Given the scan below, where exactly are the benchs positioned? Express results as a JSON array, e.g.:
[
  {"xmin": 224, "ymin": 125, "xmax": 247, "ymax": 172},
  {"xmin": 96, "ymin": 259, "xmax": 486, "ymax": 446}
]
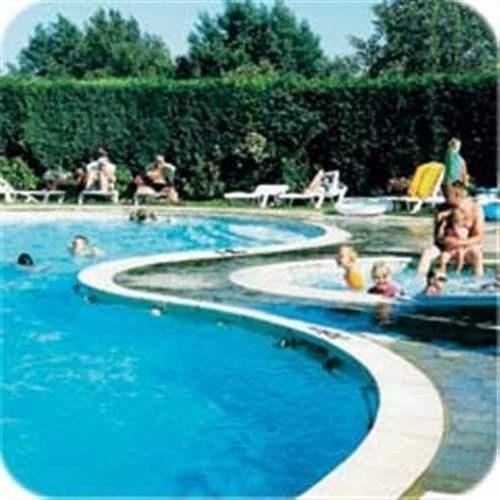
[
  {"xmin": 223, "ymin": 183, "xmax": 346, "ymax": 209},
  {"xmin": 1, "ymin": 172, "xmax": 118, "ymax": 206}
]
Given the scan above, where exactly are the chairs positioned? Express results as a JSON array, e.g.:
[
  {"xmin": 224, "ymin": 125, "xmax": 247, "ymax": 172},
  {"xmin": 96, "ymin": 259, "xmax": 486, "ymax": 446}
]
[{"xmin": 364, "ymin": 160, "xmax": 445, "ymax": 215}]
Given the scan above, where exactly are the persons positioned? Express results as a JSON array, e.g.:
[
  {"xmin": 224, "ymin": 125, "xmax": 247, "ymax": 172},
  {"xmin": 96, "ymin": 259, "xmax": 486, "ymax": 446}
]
[
  {"xmin": 415, "ymin": 179, "xmax": 486, "ymax": 275},
  {"xmin": 442, "ymin": 137, "xmax": 467, "ymax": 187},
  {"xmin": 83, "ymin": 148, "xmax": 118, "ymax": 192},
  {"xmin": 133, "ymin": 154, "xmax": 179, "ymax": 204},
  {"xmin": 129, "ymin": 208, "xmax": 158, "ymax": 224},
  {"xmin": 336, "ymin": 244, "xmax": 365, "ymax": 291},
  {"xmin": 434, "ymin": 209, "xmax": 472, "ymax": 276},
  {"xmin": 67, "ymin": 235, "xmax": 106, "ymax": 259},
  {"xmin": 420, "ymin": 266, "xmax": 449, "ymax": 298},
  {"xmin": 364, "ymin": 260, "xmax": 406, "ymax": 297}
]
